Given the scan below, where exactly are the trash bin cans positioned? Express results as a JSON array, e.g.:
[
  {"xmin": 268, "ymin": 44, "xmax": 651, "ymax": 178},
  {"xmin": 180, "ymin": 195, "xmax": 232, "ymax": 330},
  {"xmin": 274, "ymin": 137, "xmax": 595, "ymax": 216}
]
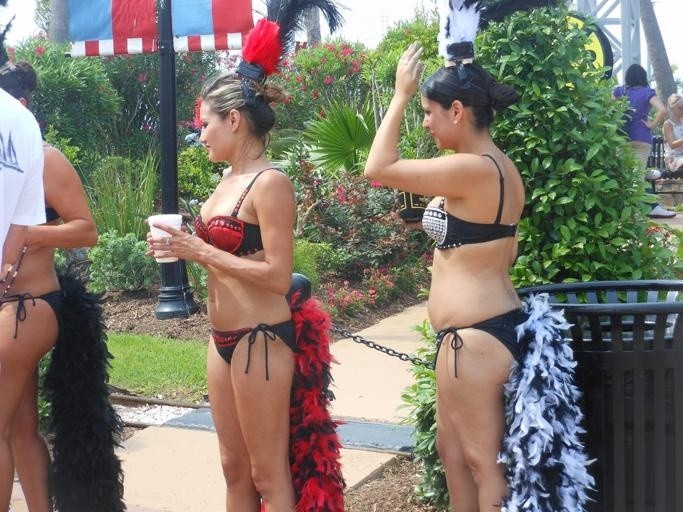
[{"xmin": 516, "ymin": 280, "xmax": 682, "ymax": 511}]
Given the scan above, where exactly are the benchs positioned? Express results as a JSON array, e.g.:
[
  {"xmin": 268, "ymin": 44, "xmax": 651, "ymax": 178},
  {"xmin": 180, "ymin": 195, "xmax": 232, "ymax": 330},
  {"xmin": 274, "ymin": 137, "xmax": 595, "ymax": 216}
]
[{"xmin": 646, "ymin": 135, "xmax": 683, "ymax": 195}]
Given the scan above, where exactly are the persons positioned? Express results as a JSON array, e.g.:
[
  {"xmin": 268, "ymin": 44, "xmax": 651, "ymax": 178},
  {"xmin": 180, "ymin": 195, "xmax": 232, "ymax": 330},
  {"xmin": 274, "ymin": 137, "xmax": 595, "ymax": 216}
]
[
  {"xmin": 361, "ymin": 41, "xmax": 530, "ymax": 512},
  {"xmin": 0, "ymin": 60, "xmax": 99, "ymax": 512},
  {"xmin": 0, "ymin": 87, "xmax": 46, "ymax": 292},
  {"xmin": 142, "ymin": 72, "xmax": 296, "ymax": 512},
  {"xmin": 610, "ymin": 63, "xmax": 664, "ymax": 196},
  {"xmin": 662, "ymin": 92, "xmax": 682, "ymax": 175}
]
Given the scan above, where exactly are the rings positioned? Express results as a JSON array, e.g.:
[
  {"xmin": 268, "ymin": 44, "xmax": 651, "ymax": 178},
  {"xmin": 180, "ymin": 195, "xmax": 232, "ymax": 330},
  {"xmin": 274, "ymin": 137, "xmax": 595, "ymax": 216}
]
[{"xmin": 165, "ymin": 236, "xmax": 171, "ymax": 247}]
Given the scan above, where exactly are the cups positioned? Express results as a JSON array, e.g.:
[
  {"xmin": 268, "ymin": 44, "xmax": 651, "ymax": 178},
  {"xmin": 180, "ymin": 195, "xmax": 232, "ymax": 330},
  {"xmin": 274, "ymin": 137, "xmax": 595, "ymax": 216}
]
[
  {"xmin": 397, "ymin": 188, "xmax": 426, "ymax": 223},
  {"xmin": 147, "ymin": 212, "xmax": 183, "ymax": 264}
]
